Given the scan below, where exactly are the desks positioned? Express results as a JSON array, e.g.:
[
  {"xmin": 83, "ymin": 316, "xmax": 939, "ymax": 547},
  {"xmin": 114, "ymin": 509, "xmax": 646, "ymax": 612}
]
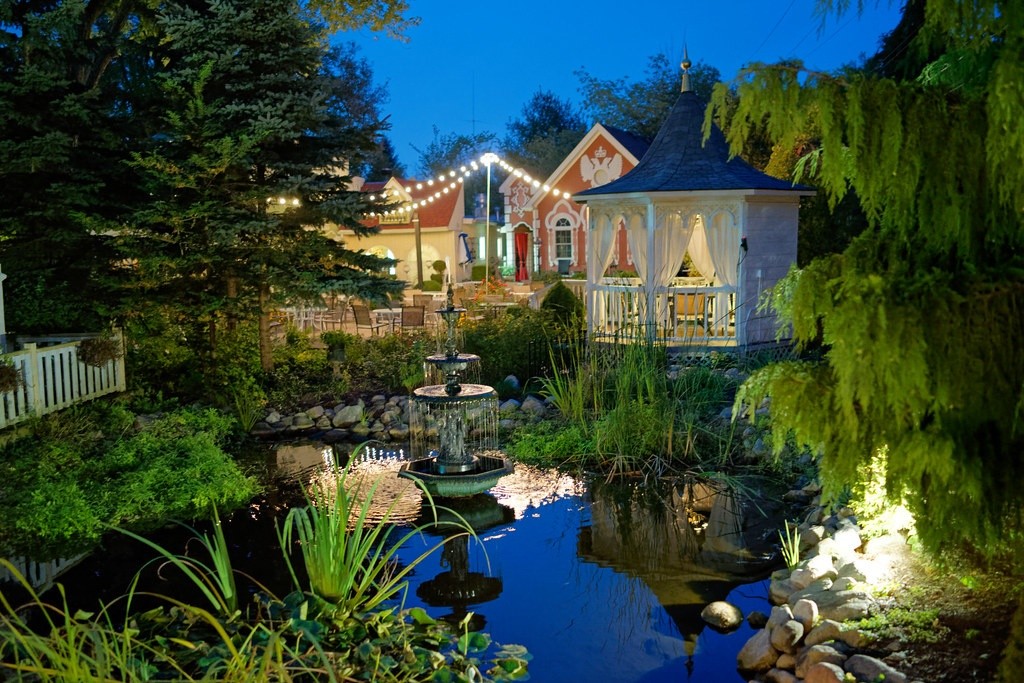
[
  {"xmin": 279, "ymin": 306, "xmax": 328, "ymax": 331},
  {"xmin": 480, "ymin": 302, "xmax": 518, "ymax": 319},
  {"xmin": 373, "ymin": 308, "xmax": 428, "ymax": 336}
]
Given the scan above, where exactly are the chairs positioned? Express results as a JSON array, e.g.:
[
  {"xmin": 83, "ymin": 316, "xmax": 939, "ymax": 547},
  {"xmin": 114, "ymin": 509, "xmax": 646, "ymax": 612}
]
[
  {"xmin": 668, "ymin": 287, "xmax": 714, "ymax": 338},
  {"xmin": 351, "ymin": 294, "xmax": 529, "ymax": 338},
  {"xmin": 269, "ymin": 294, "xmax": 355, "ymax": 336}
]
